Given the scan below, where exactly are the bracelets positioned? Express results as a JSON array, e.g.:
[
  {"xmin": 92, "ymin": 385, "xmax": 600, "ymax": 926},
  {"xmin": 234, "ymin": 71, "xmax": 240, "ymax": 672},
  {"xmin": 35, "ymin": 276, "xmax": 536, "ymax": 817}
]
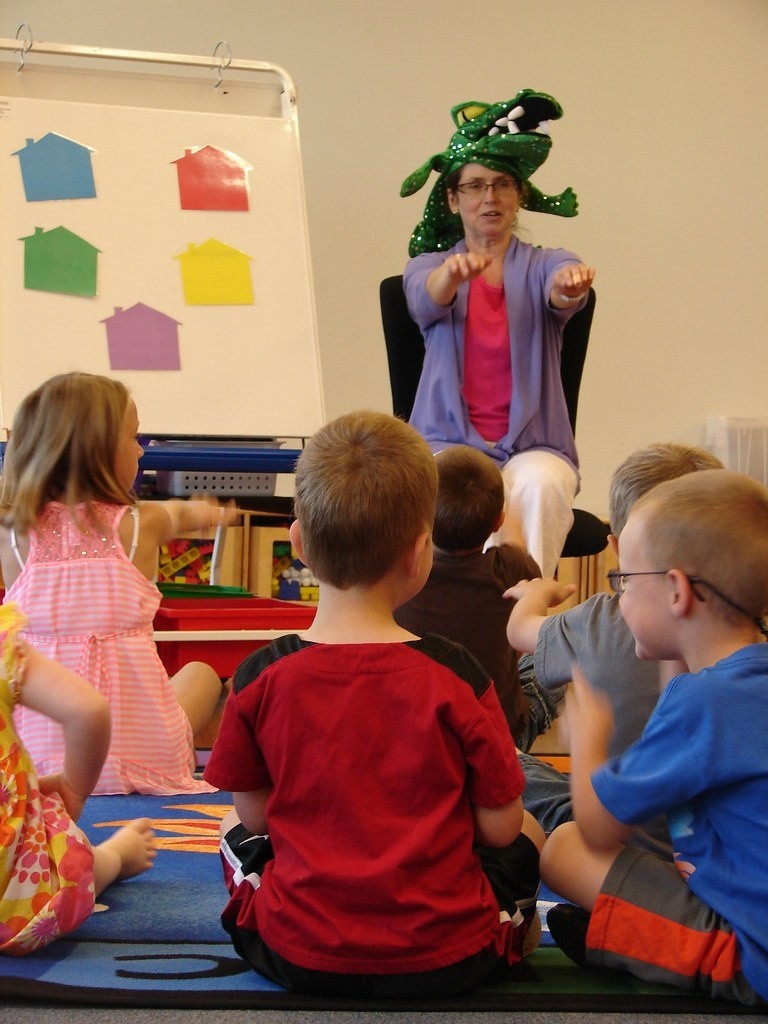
[{"xmin": 560, "ymin": 294, "xmax": 585, "ymax": 302}]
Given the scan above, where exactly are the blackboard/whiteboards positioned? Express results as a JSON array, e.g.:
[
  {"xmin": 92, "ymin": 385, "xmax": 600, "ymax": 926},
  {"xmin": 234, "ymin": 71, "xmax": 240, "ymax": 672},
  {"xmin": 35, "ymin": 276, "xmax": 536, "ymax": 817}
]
[{"xmin": 0, "ymin": 97, "xmax": 328, "ymax": 439}]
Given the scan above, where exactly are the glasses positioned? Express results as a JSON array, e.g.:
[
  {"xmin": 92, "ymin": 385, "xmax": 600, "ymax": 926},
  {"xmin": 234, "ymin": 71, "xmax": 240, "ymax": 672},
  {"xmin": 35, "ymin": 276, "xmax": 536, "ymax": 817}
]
[
  {"xmin": 452, "ymin": 178, "xmax": 520, "ymax": 194},
  {"xmin": 605, "ymin": 566, "xmax": 706, "ymax": 605}
]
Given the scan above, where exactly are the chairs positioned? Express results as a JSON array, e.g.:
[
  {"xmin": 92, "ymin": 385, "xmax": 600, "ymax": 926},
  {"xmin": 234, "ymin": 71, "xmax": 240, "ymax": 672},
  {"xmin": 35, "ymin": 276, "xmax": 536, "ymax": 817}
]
[{"xmin": 380, "ymin": 274, "xmax": 611, "ymax": 557}]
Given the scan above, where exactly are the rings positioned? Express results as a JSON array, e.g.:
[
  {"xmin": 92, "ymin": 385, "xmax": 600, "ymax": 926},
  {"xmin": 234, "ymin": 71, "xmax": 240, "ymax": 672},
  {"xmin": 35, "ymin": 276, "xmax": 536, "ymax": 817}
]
[{"xmin": 456, "ymin": 254, "xmax": 461, "ymax": 260}]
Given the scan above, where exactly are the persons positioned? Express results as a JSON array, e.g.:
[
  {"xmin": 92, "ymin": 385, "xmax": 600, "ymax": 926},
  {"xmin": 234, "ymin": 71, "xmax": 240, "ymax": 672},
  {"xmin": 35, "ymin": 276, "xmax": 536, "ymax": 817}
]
[
  {"xmin": 401, "ymin": 157, "xmax": 595, "ymax": 580},
  {"xmin": 216, "ymin": 408, "xmax": 546, "ymax": 1012},
  {"xmin": 503, "ymin": 444, "xmax": 768, "ymax": 1012},
  {"xmin": 1, "ymin": 373, "xmax": 238, "ymax": 957}
]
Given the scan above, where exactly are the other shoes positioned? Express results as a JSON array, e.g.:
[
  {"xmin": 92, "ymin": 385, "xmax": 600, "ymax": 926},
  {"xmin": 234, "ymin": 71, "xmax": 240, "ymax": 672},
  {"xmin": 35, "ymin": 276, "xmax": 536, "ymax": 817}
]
[{"xmin": 546, "ymin": 902, "xmax": 634, "ymax": 986}]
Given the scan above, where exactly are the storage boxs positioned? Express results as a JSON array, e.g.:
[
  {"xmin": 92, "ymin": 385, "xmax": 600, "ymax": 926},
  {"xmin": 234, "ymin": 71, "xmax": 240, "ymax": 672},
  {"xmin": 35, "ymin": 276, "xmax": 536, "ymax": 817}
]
[
  {"xmin": 154, "ymin": 440, "xmax": 287, "ymax": 496},
  {"xmin": 154, "ymin": 595, "xmax": 319, "ymax": 678},
  {"xmin": 157, "ymin": 526, "xmax": 320, "ymax": 605}
]
